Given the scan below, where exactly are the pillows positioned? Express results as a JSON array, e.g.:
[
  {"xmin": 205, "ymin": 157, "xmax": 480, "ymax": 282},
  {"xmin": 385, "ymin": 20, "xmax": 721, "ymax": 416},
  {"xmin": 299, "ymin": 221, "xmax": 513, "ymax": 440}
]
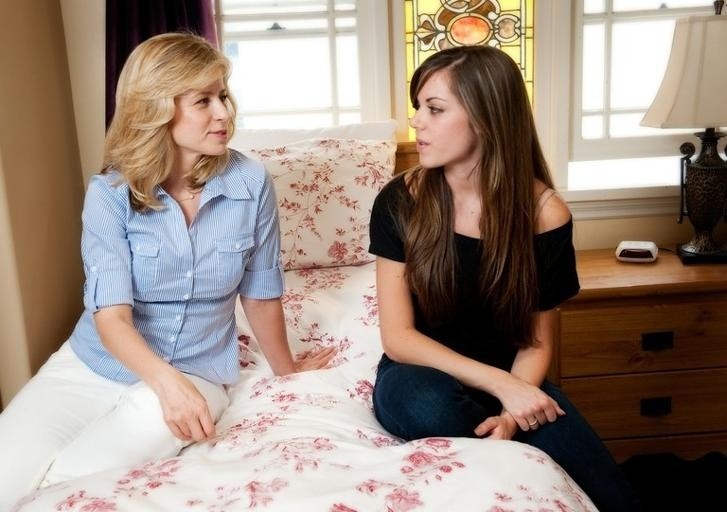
[{"xmin": 244, "ymin": 134, "xmax": 401, "ymax": 275}]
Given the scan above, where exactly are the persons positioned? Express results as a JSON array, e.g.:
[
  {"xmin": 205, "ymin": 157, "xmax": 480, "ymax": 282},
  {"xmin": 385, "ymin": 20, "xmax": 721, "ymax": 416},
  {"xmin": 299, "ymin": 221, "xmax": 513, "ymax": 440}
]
[
  {"xmin": 367, "ymin": 45, "xmax": 653, "ymax": 512},
  {"xmin": 0, "ymin": 33, "xmax": 337, "ymax": 512}
]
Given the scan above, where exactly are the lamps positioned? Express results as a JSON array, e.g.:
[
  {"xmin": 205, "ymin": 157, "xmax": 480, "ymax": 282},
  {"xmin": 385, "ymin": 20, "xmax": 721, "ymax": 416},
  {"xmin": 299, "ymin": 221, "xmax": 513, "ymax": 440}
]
[{"xmin": 630, "ymin": 0, "xmax": 727, "ymax": 267}]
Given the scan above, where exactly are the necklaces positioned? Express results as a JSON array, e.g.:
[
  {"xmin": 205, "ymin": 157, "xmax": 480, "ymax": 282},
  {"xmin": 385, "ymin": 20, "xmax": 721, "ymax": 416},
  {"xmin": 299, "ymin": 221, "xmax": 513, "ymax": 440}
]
[{"xmin": 160, "ymin": 182, "xmax": 195, "ymax": 203}]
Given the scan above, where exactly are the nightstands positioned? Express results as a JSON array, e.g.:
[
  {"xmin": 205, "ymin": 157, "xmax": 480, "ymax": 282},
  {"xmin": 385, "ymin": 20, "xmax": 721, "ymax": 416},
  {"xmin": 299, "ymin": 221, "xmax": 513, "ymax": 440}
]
[{"xmin": 541, "ymin": 241, "xmax": 726, "ymax": 467}]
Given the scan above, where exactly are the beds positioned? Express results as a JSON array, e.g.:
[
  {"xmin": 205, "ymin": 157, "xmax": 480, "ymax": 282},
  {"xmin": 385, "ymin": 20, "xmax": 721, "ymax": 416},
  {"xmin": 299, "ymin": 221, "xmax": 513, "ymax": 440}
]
[{"xmin": 51, "ymin": 140, "xmax": 593, "ymax": 512}]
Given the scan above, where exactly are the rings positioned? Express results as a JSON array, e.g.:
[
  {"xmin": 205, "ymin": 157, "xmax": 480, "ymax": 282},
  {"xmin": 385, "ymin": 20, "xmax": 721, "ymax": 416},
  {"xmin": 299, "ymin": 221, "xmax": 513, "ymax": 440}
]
[{"xmin": 529, "ymin": 419, "xmax": 538, "ymax": 426}]
